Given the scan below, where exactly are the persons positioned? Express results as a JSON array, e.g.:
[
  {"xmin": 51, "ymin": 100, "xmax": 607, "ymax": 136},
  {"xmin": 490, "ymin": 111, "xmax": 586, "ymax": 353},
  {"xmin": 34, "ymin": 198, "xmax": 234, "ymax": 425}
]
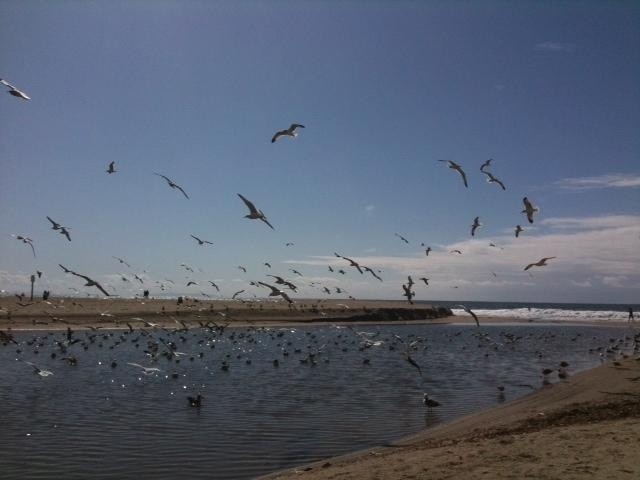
[{"xmin": 628, "ymin": 306, "xmax": 635, "ymax": 322}]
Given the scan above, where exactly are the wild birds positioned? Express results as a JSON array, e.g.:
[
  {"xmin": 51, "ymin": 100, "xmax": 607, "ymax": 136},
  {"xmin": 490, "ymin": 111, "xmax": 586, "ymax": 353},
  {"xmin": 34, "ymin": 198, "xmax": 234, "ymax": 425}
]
[
  {"xmin": 0, "ymin": 158, "xmax": 640, "ymax": 412},
  {"xmin": 0, "ymin": 79, "xmax": 31, "ymax": 100},
  {"xmin": 271, "ymin": 124, "xmax": 305, "ymax": 143}
]
